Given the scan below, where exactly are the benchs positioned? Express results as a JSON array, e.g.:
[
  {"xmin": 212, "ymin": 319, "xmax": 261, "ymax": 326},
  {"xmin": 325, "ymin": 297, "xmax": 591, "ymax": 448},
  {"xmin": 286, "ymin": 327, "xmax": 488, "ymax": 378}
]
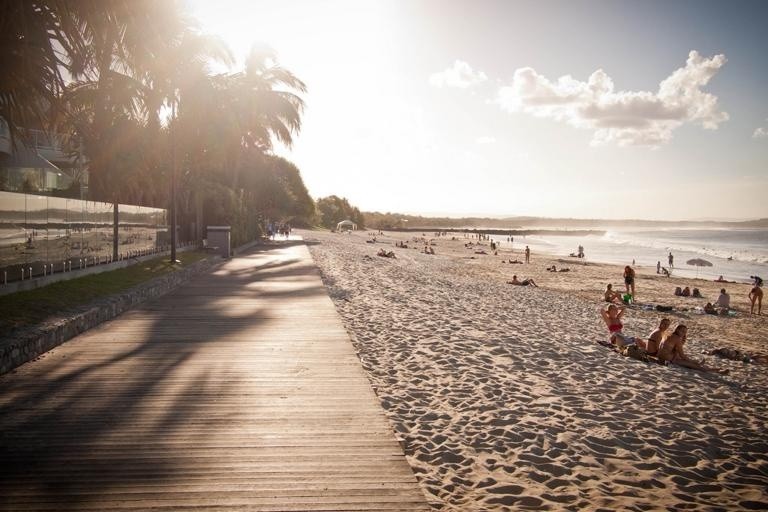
[{"xmin": 201, "ymin": 238, "xmax": 219, "ymax": 251}]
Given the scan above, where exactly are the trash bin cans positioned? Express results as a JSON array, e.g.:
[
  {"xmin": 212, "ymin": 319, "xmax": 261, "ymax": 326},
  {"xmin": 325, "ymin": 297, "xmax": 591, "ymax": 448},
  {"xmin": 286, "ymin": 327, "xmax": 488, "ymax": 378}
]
[{"xmin": 207, "ymin": 226, "xmax": 231, "ymax": 258}]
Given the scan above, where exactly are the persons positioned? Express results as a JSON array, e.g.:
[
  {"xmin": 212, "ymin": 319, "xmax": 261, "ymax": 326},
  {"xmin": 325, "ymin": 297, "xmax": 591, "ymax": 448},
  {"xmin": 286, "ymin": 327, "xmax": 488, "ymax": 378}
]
[
  {"xmin": 330, "ymin": 219, "xmax": 586, "ymax": 288},
  {"xmin": 603, "ymin": 250, "xmax": 765, "ymax": 315},
  {"xmin": 600, "ymin": 303, "xmax": 646, "ymax": 353},
  {"xmin": 645, "ymin": 318, "xmax": 670, "ymax": 357},
  {"xmin": 657, "ymin": 324, "xmax": 707, "ymax": 372},
  {"xmin": 257, "ymin": 216, "xmax": 292, "ymax": 244}
]
